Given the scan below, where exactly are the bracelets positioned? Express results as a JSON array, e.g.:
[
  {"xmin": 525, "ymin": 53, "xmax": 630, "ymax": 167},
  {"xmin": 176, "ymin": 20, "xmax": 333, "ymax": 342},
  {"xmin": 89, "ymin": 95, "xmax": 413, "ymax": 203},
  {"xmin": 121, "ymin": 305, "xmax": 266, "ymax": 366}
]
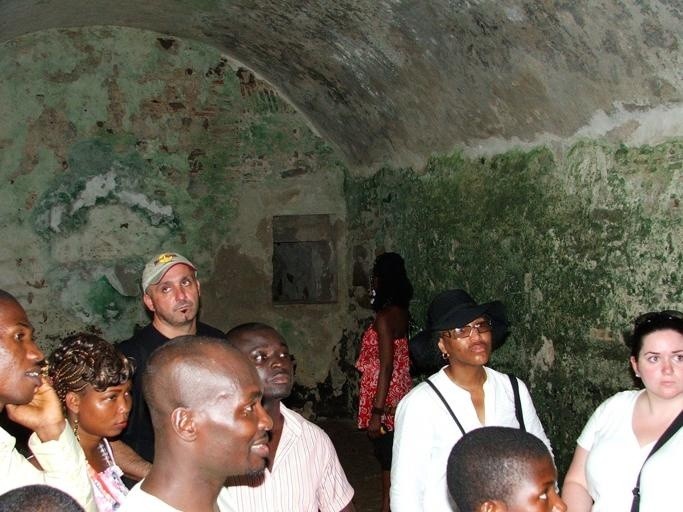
[{"xmin": 372, "ymin": 406, "xmax": 385, "ymax": 415}]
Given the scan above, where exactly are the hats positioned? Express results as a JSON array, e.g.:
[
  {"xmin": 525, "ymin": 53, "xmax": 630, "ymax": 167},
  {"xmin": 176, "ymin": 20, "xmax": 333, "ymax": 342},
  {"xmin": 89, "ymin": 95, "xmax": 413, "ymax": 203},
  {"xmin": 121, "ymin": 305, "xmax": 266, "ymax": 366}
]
[
  {"xmin": 141, "ymin": 253, "xmax": 198, "ymax": 294},
  {"xmin": 407, "ymin": 289, "xmax": 507, "ymax": 375}
]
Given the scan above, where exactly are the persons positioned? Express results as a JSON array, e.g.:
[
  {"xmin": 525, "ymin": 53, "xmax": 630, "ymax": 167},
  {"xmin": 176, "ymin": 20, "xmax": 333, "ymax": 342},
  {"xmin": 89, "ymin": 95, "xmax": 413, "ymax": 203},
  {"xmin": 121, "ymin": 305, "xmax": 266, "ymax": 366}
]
[
  {"xmin": 389, "ymin": 289, "xmax": 559, "ymax": 512},
  {"xmin": 447, "ymin": 425, "xmax": 569, "ymax": 512},
  {"xmin": 353, "ymin": 251, "xmax": 414, "ymax": 512},
  {"xmin": 559, "ymin": 310, "xmax": 683, "ymax": 512}
]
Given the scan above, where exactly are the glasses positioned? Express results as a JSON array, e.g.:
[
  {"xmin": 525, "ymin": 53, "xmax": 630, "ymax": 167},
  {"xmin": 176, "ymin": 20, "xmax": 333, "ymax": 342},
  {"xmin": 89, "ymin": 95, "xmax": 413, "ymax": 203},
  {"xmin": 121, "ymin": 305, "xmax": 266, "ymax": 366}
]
[
  {"xmin": 438, "ymin": 319, "xmax": 492, "ymax": 338},
  {"xmin": 634, "ymin": 310, "xmax": 683, "ymax": 335}
]
